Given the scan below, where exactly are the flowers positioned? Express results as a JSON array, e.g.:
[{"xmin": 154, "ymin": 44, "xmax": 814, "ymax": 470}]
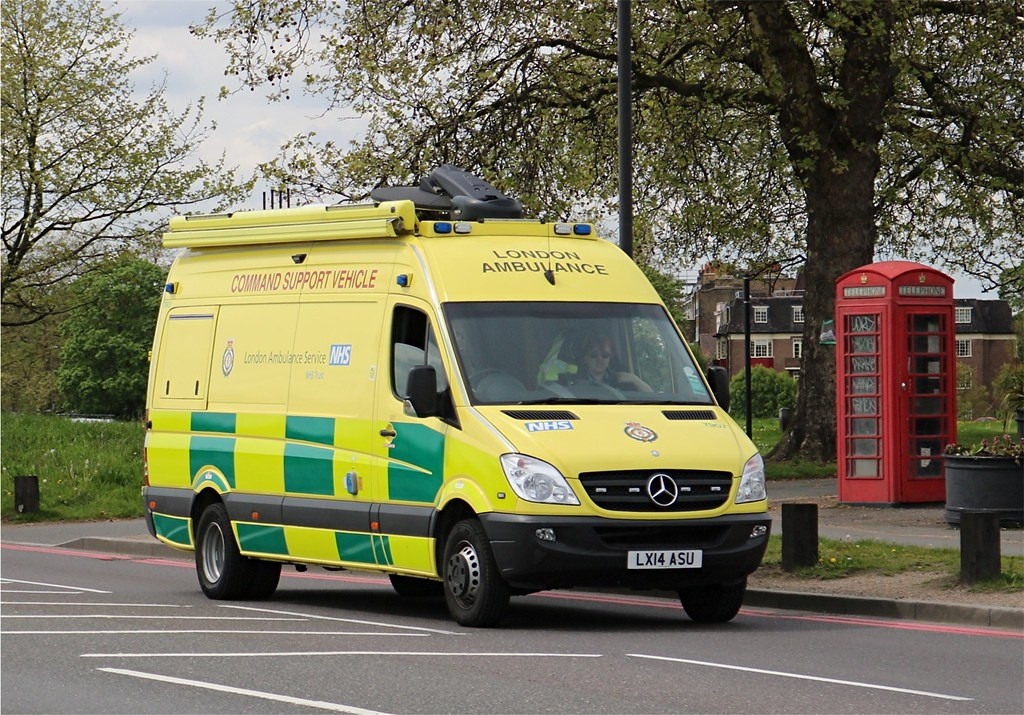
[{"xmin": 943, "ymin": 434, "xmax": 1024, "ymax": 464}]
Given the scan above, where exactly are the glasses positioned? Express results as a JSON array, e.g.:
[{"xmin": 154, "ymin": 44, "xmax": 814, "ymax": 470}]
[{"xmin": 591, "ymin": 351, "xmax": 612, "ymax": 359}]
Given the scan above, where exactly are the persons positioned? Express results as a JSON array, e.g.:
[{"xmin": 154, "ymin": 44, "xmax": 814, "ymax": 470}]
[
  {"xmin": 451, "ymin": 319, "xmax": 476, "ymax": 377},
  {"xmin": 568, "ymin": 334, "xmax": 654, "ymax": 392}
]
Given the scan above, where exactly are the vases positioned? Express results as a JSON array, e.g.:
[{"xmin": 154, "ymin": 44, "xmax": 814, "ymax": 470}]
[{"xmin": 941, "ymin": 453, "xmax": 1024, "ymax": 529}]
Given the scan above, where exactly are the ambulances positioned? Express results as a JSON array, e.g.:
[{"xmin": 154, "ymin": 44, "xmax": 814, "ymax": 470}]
[{"xmin": 139, "ymin": 166, "xmax": 774, "ymax": 627}]
